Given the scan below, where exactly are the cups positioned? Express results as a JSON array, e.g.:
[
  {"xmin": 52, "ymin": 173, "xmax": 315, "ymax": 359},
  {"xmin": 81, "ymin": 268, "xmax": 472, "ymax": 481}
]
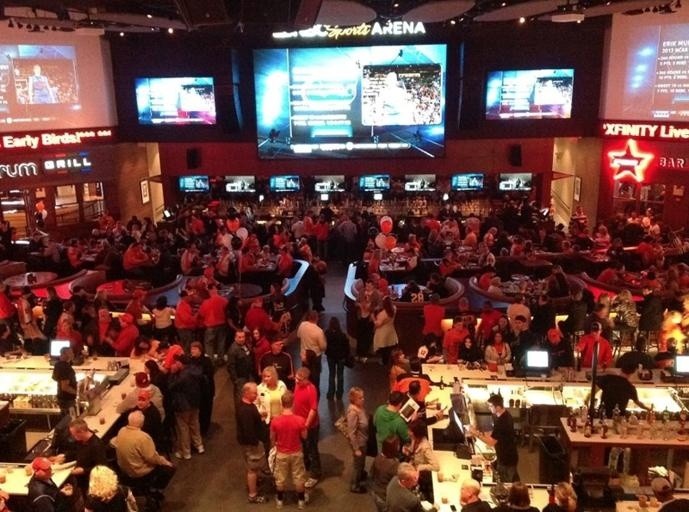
[{"xmin": 639, "ymin": 494, "xmax": 658, "ymax": 507}]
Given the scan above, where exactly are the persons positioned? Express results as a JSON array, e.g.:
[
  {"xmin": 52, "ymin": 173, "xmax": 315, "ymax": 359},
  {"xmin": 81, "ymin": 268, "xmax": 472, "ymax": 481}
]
[
  {"xmin": 26, "ymin": 63, "xmax": 54, "ymax": 105},
  {"xmin": 1, "ymin": 200, "xmax": 350, "ymax": 510},
  {"xmin": 346, "ymin": 199, "xmax": 689, "ymax": 512}
]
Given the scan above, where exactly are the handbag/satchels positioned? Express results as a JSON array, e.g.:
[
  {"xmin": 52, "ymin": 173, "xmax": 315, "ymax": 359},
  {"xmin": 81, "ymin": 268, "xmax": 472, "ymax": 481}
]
[
  {"xmin": 345, "ymin": 354, "xmax": 355, "ymax": 368},
  {"xmin": 333, "ymin": 415, "xmax": 358, "ymax": 440}
]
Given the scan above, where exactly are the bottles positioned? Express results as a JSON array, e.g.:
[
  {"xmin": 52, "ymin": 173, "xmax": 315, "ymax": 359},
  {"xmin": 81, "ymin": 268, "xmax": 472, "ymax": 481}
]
[
  {"xmin": 440, "ymin": 377, "xmax": 444, "ymax": 391},
  {"xmin": 549, "ymin": 484, "xmax": 555, "ymax": 504},
  {"xmin": 565, "ymin": 404, "xmax": 670, "ymax": 440}
]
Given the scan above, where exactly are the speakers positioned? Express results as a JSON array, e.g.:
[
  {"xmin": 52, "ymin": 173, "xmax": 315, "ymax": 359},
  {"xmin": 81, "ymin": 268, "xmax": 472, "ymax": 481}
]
[
  {"xmin": 509, "ymin": 144, "xmax": 521, "ymax": 164},
  {"xmin": 185, "ymin": 149, "xmax": 199, "ymax": 169}
]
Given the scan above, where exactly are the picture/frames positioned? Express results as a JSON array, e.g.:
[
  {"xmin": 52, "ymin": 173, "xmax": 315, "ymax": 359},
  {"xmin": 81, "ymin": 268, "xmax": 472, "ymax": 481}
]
[
  {"xmin": 139, "ymin": 179, "xmax": 151, "ymax": 205},
  {"xmin": 573, "ymin": 176, "xmax": 582, "ymax": 202}
]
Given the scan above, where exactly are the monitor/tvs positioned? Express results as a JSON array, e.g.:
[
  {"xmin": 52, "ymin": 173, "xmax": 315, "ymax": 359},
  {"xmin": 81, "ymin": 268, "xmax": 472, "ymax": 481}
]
[
  {"xmin": 498, "ymin": 173, "xmax": 534, "ymax": 192},
  {"xmin": 404, "ymin": 173, "xmax": 435, "ymax": 192},
  {"xmin": 672, "ymin": 354, "xmax": 689, "ymax": 376},
  {"xmin": 526, "ymin": 350, "xmax": 551, "ymax": 369},
  {"xmin": 225, "ymin": 174, "xmax": 255, "ymax": 195},
  {"xmin": 269, "ymin": 175, "xmax": 302, "ymax": 193},
  {"xmin": 450, "ymin": 173, "xmax": 485, "ymax": 193},
  {"xmin": 311, "ymin": 174, "xmax": 348, "ymax": 193},
  {"xmin": 449, "ymin": 410, "xmax": 464, "ymax": 440},
  {"xmin": 47, "ymin": 340, "xmax": 71, "ymax": 358},
  {"xmin": 357, "ymin": 174, "xmax": 392, "ymax": 190},
  {"xmin": 180, "ymin": 175, "xmax": 209, "ymax": 193}
]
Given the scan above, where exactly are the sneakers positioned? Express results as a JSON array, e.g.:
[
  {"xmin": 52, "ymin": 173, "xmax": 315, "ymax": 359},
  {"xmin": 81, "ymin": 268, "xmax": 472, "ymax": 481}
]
[
  {"xmin": 351, "ymin": 472, "xmax": 368, "ymax": 493},
  {"xmin": 175, "ymin": 452, "xmax": 191, "ymax": 460},
  {"xmin": 197, "ymin": 445, "xmax": 205, "ymax": 454},
  {"xmin": 248, "ymin": 469, "xmax": 318, "ymax": 509}
]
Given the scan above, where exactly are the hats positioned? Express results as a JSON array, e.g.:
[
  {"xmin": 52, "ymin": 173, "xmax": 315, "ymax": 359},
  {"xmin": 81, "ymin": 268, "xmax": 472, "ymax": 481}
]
[
  {"xmin": 174, "ymin": 354, "xmax": 190, "ymax": 365},
  {"xmin": 138, "ymin": 390, "xmax": 149, "ymax": 400},
  {"xmin": 652, "ymin": 477, "xmax": 673, "ymax": 493}
]
